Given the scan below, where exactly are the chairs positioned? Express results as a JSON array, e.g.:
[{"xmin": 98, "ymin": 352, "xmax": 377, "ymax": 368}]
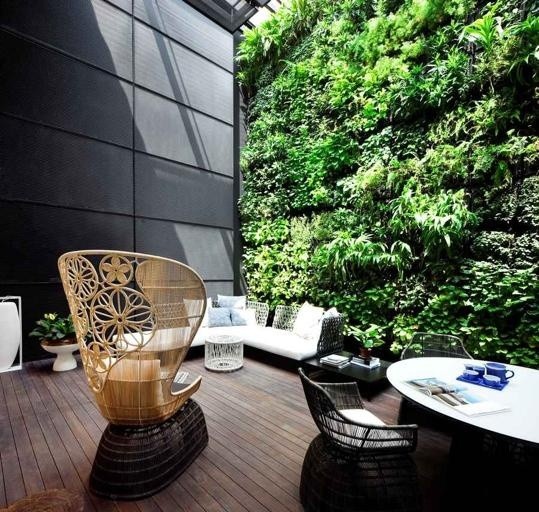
[
  {"xmin": 399, "ymin": 332, "xmax": 474, "ymax": 360},
  {"xmin": 56, "ymin": 248, "xmax": 210, "ymax": 500},
  {"xmin": 297, "ymin": 367, "xmax": 421, "ymax": 512}
]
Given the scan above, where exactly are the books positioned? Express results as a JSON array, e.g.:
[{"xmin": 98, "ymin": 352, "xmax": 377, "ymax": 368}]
[
  {"xmin": 406, "ymin": 376, "xmax": 509, "ymax": 418},
  {"xmin": 320, "ymin": 354, "xmax": 381, "ymax": 370}
]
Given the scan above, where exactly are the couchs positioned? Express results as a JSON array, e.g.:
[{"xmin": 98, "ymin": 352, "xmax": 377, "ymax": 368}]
[{"xmin": 113, "ymin": 296, "xmax": 346, "ymax": 376}]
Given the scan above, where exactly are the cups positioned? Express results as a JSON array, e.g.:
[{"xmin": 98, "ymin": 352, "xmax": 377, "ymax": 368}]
[{"xmin": 462, "ymin": 362, "xmax": 514, "ymax": 387}]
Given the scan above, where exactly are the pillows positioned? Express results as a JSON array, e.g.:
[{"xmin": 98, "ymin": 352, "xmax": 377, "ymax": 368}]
[
  {"xmin": 291, "ymin": 300, "xmax": 338, "ymax": 345},
  {"xmin": 181, "ymin": 294, "xmax": 257, "ymax": 328}
]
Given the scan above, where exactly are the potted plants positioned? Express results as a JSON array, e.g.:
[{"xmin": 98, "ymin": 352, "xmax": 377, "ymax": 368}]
[{"xmin": 350, "ymin": 329, "xmax": 385, "ymax": 359}]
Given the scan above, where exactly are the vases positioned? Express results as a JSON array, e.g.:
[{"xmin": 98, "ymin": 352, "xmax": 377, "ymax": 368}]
[{"xmin": 39, "ymin": 337, "xmax": 81, "ymax": 371}]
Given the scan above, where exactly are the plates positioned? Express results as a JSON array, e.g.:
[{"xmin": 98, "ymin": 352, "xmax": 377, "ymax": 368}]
[{"xmin": 456, "ymin": 375, "xmax": 510, "ymax": 391}]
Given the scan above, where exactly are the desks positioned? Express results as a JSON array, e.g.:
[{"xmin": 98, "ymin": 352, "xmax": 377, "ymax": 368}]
[
  {"xmin": 385, "ymin": 356, "xmax": 539, "ymax": 510},
  {"xmin": 306, "ymin": 351, "xmax": 395, "ymax": 401}
]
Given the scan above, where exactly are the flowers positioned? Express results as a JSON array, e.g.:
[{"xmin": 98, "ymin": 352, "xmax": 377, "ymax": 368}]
[{"xmin": 28, "ymin": 312, "xmax": 93, "ymax": 341}]
